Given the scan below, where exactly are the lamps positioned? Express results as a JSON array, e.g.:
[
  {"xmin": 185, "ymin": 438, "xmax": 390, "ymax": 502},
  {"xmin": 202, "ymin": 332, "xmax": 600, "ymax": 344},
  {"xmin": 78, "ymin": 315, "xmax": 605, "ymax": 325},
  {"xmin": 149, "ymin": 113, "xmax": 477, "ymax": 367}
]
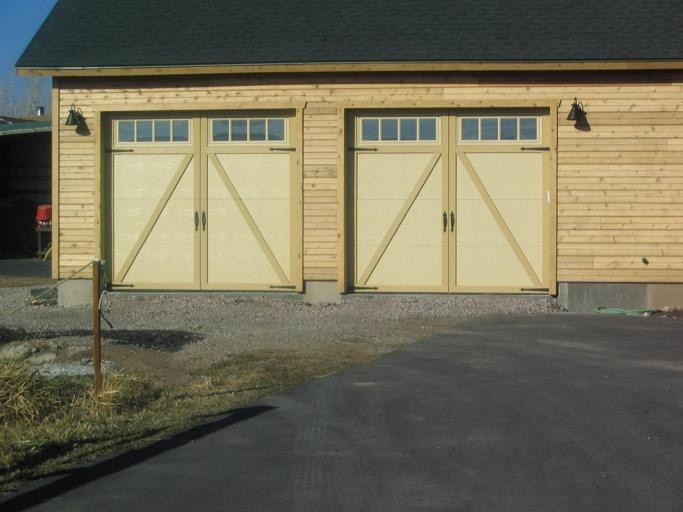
[
  {"xmin": 66, "ymin": 104, "xmax": 85, "ymax": 128},
  {"xmin": 567, "ymin": 97, "xmax": 586, "ymax": 122}
]
